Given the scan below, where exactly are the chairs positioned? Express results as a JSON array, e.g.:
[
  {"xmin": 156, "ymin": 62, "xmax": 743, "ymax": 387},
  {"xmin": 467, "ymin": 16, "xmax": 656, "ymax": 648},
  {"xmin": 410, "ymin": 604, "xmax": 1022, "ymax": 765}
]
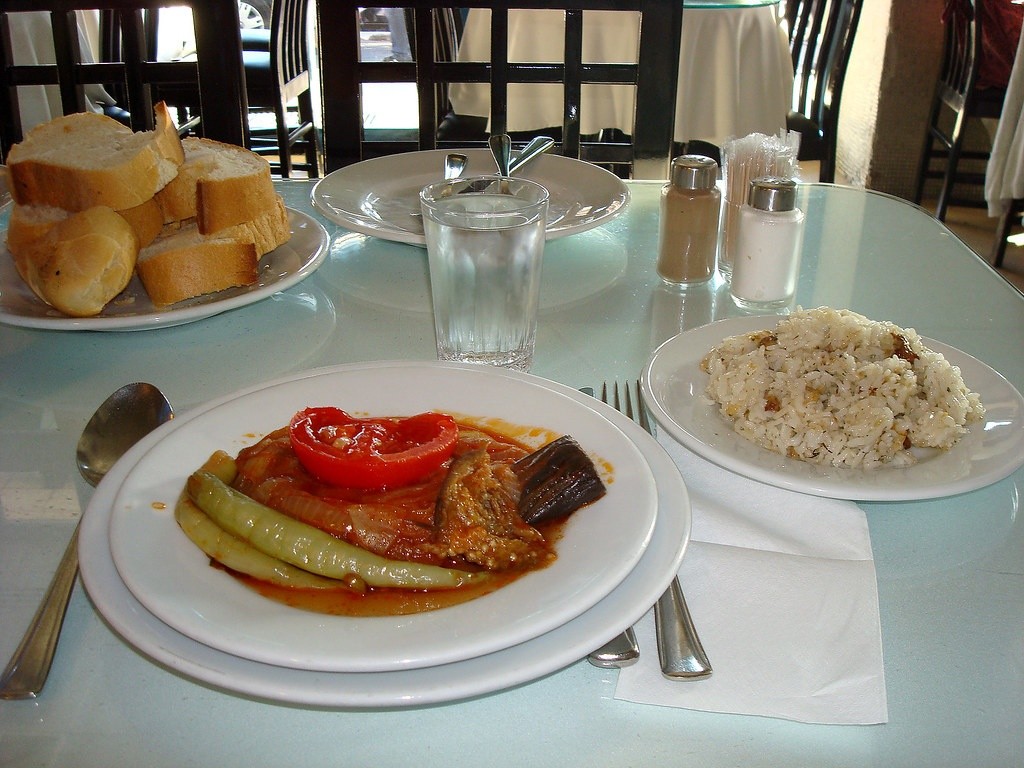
[
  {"xmin": 914, "ymin": 0, "xmax": 1018, "ymax": 222},
  {"xmin": 175, "ymin": 0, "xmax": 321, "ymax": 183},
  {"xmin": 669, "ymin": 0, "xmax": 863, "ymax": 185},
  {"xmin": 322, "ymin": 0, "xmax": 687, "ymax": 178}
]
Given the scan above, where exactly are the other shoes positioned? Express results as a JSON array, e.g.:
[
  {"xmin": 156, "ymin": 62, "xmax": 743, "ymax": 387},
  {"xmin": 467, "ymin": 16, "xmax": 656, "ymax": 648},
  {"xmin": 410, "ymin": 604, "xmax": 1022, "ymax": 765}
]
[{"xmin": 384, "ymin": 56, "xmax": 398, "ymax": 62}]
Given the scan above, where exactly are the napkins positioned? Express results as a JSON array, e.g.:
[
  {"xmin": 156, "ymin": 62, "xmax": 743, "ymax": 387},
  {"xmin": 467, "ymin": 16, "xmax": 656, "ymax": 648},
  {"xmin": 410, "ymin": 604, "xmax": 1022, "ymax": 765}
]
[{"xmin": 613, "ymin": 419, "xmax": 891, "ymax": 726}]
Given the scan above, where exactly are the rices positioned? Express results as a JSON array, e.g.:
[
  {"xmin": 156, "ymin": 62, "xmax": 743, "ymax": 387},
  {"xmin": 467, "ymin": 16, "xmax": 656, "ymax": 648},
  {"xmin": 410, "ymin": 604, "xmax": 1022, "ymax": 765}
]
[{"xmin": 700, "ymin": 306, "xmax": 986, "ymax": 469}]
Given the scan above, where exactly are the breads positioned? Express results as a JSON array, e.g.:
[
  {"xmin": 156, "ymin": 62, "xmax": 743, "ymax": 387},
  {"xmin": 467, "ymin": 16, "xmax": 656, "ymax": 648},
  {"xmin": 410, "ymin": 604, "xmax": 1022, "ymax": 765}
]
[{"xmin": 5, "ymin": 103, "xmax": 291, "ymax": 317}]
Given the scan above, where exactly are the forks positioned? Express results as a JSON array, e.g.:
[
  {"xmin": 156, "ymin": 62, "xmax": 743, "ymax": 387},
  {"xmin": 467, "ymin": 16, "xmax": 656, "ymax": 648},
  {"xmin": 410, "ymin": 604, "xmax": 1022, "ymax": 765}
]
[{"xmin": 597, "ymin": 376, "xmax": 713, "ymax": 681}]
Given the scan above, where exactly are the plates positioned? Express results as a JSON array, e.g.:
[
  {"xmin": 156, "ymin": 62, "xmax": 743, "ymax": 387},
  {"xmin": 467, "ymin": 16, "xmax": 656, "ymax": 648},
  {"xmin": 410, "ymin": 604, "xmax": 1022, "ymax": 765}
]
[
  {"xmin": 108, "ymin": 364, "xmax": 657, "ymax": 671},
  {"xmin": 309, "ymin": 149, "xmax": 632, "ymax": 248},
  {"xmin": 640, "ymin": 314, "xmax": 1024, "ymax": 498},
  {"xmin": 77, "ymin": 362, "xmax": 693, "ymax": 709},
  {"xmin": 0, "ymin": 207, "xmax": 331, "ymax": 332}
]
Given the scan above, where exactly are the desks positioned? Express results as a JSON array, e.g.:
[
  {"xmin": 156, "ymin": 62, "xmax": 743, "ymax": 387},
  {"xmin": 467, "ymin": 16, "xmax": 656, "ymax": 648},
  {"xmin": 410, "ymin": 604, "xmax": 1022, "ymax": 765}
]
[{"xmin": 451, "ymin": 0, "xmax": 795, "ymax": 153}]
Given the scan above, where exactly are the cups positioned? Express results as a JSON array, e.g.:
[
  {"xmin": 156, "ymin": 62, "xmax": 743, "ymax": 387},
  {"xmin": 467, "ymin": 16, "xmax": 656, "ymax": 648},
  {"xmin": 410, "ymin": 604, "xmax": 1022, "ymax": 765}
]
[
  {"xmin": 419, "ymin": 174, "xmax": 550, "ymax": 373},
  {"xmin": 717, "ymin": 195, "xmax": 740, "ymax": 284}
]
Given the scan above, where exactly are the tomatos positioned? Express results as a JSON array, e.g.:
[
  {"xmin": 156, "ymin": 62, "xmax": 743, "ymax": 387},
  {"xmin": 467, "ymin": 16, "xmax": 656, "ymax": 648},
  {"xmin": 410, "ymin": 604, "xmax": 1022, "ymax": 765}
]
[{"xmin": 288, "ymin": 406, "xmax": 459, "ymax": 490}]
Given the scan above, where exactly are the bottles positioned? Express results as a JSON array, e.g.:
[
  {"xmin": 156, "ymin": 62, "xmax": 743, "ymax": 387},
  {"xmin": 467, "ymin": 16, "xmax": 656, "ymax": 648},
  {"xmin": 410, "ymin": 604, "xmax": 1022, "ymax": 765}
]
[
  {"xmin": 727, "ymin": 175, "xmax": 805, "ymax": 311},
  {"xmin": 654, "ymin": 154, "xmax": 721, "ymax": 289}
]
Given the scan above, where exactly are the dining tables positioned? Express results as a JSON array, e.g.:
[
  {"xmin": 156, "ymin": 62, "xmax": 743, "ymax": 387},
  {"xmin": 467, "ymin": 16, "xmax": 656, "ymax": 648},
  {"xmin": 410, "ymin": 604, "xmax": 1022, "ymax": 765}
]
[{"xmin": 0, "ymin": 184, "xmax": 1024, "ymax": 768}]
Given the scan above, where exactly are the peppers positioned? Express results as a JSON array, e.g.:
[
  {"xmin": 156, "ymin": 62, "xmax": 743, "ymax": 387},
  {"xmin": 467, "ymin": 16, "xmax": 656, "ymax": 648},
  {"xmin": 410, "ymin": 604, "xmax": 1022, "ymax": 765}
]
[{"xmin": 175, "ymin": 448, "xmax": 495, "ymax": 593}]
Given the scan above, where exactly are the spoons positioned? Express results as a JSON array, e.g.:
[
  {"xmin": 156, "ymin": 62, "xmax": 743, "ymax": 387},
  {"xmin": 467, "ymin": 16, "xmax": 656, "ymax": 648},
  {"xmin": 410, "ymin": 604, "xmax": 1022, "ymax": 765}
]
[{"xmin": 0, "ymin": 380, "xmax": 173, "ymax": 700}]
